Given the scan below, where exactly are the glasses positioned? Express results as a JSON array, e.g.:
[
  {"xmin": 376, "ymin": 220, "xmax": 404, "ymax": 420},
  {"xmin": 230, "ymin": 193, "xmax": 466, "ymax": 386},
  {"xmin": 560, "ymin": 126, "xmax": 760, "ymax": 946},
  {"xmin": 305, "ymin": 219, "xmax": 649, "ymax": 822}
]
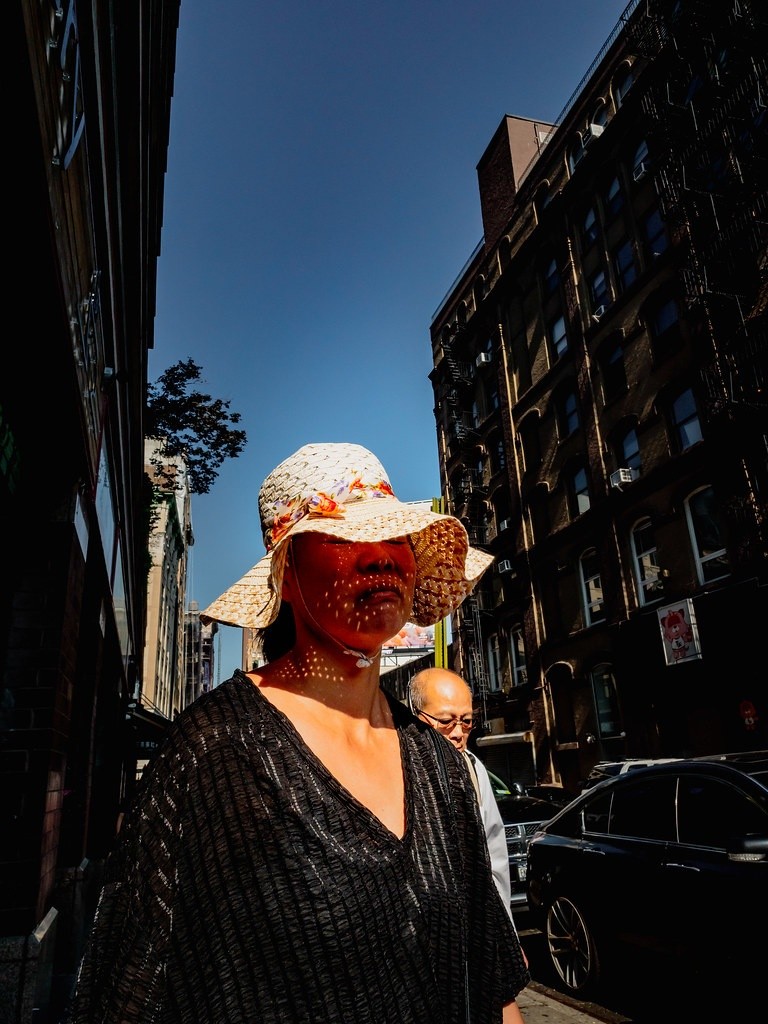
[{"xmin": 414, "ymin": 702, "xmax": 477, "ymax": 732}]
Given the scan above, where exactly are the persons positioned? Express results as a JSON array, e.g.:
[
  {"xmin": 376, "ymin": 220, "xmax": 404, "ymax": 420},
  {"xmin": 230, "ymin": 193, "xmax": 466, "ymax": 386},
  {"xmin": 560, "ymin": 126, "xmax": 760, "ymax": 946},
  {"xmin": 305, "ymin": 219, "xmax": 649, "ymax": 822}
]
[{"xmin": 65, "ymin": 442, "xmax": 531, "ymax": 1024}]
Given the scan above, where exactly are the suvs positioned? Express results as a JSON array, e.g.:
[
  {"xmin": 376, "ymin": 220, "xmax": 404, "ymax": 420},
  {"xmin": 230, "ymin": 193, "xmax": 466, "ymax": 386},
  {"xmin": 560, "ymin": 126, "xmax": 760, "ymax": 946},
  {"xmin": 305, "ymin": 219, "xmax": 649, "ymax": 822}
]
[{"xmin": 581, "ymin": 756, "xmax": 768, "ymax": 802}]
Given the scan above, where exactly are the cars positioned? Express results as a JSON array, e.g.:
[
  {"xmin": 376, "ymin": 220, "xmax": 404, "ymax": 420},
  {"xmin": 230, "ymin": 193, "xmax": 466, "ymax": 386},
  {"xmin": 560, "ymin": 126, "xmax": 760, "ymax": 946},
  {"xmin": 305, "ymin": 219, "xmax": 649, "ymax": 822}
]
[
  {"xmin": 483, "ymin": 763, "xmax": 584, "ymax": 916},
  {"xmin": 527, "ymin": 754, "xmax": 768, "ymax": 1014}
]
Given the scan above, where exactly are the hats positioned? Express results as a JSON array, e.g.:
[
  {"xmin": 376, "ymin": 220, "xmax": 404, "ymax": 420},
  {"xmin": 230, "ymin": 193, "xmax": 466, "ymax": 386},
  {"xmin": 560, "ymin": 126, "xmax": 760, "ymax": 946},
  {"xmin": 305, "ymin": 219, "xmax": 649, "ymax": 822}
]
[{"xmin": 201, "ymin": 441, "xmax": 495, "ymax": 631}]
